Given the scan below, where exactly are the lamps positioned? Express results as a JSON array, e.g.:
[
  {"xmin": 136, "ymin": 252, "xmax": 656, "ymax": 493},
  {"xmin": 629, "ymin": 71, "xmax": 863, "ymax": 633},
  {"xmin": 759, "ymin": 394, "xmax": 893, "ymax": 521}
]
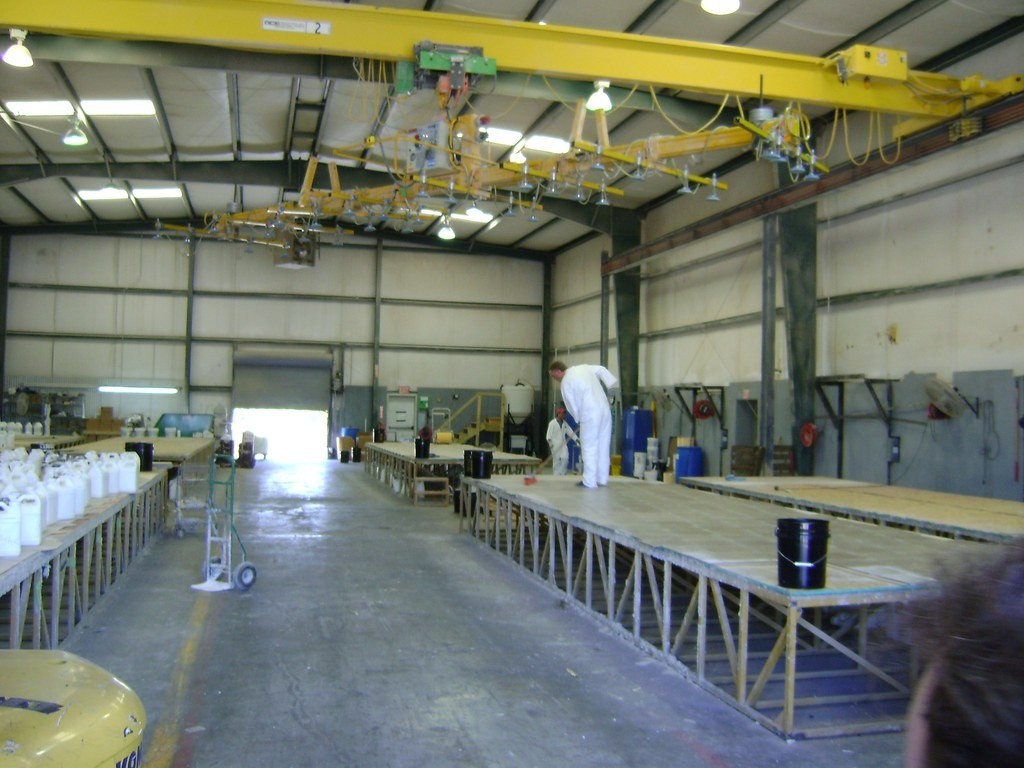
[
  {"xmin": 155, "ymin": 126, "xmax": 827, "ymax": 255},
  {"xmin": 2, "ymin": 29, "xmax": 33, "ymax": 67},
  {"xmin": 587, "ymin": 81, "xmax": 613, "ymax": 112},
  {"xmin": 98, "ymin": 386, "xmax": 181, "ymax": 394},
  {"xmin": 63, "ymin": 112, "xmax": 88, "ymax": 146}
]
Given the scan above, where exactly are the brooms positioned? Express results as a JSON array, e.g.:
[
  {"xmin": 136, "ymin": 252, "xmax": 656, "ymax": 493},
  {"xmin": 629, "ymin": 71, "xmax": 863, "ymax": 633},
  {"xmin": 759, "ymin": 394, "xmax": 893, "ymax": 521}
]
[{"xmin": 521, "ymin": 425, "xmax": 582, "ymax": 487}]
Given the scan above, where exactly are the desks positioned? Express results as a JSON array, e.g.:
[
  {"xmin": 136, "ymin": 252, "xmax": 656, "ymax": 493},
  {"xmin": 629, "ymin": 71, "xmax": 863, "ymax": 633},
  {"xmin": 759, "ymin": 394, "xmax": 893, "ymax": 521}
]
[
  {"xmin": 59, "ymin": 437, "xmax": 217, "ymax": 508},
  {"xmin": 0, "ymin": 468, "xmax": 166, "ymax": 649},
  {"xmin": 460, "ymin": 474, "xmax": 1007, "ymax": 740},
  {"xmin": 364, "ymin": 442, "xmax": 540, "ymax": 507},
  {"xmin": 678, "ymin": 477, "xmax": 1024, "ymax": 547}
]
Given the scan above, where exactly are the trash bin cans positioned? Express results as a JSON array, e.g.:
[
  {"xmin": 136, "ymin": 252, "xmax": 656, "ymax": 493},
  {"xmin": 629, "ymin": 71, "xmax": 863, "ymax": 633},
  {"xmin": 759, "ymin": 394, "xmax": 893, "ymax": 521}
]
[
  {"xmin": 342, "ymin": 426, "xmax": 360, "ymax": 446},
  {"xmin": 675, "ymin": 446, "xmax": 703, "ymax": 489}
]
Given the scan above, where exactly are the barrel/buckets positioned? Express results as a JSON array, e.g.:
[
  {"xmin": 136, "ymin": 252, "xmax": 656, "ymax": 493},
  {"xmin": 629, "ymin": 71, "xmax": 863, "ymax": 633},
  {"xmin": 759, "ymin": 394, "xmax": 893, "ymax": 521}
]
[
  {"xmin": 373, "ymin": 428, "xmax": 384, "ymax": 442},
  {"xmin": 775, "ymin": 518, "xmax": 829, "ymax": 590},
  {"xmin": 336, "ymin": 436, "xmax": 354, "ymax": 461},
  {"xmin": 120, "ymin": 427, "xmax": 176, "ymax": 439},
  {"xmin": 463, "ymin": 451, "xmax": 492, "ymax": 479},
  {"xmin": 353, "ymin": 448, "xmax": 361, "ymax": 462},
  {"xmin": 414, "ymin": 440, "xmax": 428, "ymax": 458},
  {"xmin": 454, "ymin": 491, "xmax": 476, "ymax": 517},
  {"xmin": 126, "ymin": 443, "xmax": 153, "ymax": 471},
  {"xmin": 341, "ymin": 451, "xmax": 349, "ymax": 463},
  {"xmin": 356, "ymin": 432, "xmax": 371, "ymax": 447},
  {"xmin": 342, "ymin": 428, "xmax": 359, "ymax": 441}
]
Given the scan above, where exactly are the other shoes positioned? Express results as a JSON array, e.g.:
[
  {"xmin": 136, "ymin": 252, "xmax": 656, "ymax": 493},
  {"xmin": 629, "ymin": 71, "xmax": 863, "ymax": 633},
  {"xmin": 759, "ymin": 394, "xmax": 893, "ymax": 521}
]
[{"xmin": 577, "ymin": 481, "xmax": 588, "ymax": 488}]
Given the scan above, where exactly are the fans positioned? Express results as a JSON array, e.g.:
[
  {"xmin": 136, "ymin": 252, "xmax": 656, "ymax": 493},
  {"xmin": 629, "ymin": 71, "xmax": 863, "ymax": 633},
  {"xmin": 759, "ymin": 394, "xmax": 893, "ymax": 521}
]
[{"xmin": 923, "ymin": 377, "xmax": 980, "ymax": 420}]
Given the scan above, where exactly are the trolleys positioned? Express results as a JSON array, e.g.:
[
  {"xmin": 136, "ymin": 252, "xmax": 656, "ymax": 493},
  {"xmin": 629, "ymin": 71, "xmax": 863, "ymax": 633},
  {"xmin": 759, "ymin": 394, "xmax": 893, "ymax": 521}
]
[{"xmin": 187, "ymin": 450, "xmax": 258, "ymax": 593}]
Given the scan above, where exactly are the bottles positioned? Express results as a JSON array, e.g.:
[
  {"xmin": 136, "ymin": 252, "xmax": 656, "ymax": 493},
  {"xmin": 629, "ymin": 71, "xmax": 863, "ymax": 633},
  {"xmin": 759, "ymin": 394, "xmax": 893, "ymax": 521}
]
[
  {"xmin": 177, "ymin": 430, "xmax": 181, "ymax": 438},
  {"xmin": 170, "ymin": 474, "xmax": 180, "ymax": 500},
  {"xmin": 0, "ymin": 447, "xmax": 139, "ymax": 557},
  {"xmin": 370, "ymin": 461, "xmax": 425, "ymax": 500},
  {"xmin": 0, "ymin": 421, "xmax": 43, "ymax": 448}
]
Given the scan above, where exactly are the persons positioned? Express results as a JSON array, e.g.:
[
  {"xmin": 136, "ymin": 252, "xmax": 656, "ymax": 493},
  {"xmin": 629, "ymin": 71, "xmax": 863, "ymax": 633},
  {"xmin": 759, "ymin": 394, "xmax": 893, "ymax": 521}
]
[
  {"xmin": 546, "ymin": 408, "xmax": 580, "ymax": 475},
  {"xmin": 549, "ymin": 361, "xmax": 618, "ymax": 488},
  {"xmin": 903, "ymin": 535, "xmax": 1024, "ymax": 768}
]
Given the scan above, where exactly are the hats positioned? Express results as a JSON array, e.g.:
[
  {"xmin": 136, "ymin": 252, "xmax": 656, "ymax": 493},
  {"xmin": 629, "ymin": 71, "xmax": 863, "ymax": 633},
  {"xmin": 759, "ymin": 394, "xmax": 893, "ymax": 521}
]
[{"xmin": 557, "ymin": 408, "xmax": 566, "ymax": 413}]
